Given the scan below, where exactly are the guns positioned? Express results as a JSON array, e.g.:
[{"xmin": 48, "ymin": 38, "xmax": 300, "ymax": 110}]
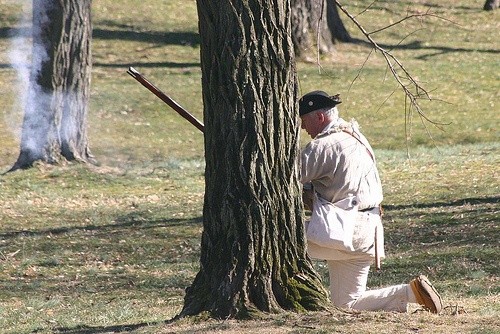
[{"xmin": 126, "ymin": 66, "xmax": 204, "ymax": 133}]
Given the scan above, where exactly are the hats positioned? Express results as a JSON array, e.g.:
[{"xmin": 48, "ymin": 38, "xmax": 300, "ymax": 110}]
[{"xmin": 296, "ymin": 89, "xmax": 340, "ymax": 118}]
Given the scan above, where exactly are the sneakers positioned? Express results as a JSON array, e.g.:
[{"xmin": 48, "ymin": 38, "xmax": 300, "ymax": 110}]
[{"xmin": 411, "ymin": 275, "xmax": 443, "ymax": 314}]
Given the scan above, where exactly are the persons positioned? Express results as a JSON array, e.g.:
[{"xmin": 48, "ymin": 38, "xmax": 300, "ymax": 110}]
[{"xmin": 296, "ymin": 89, "xmax": 445, "ymax": 316}]
